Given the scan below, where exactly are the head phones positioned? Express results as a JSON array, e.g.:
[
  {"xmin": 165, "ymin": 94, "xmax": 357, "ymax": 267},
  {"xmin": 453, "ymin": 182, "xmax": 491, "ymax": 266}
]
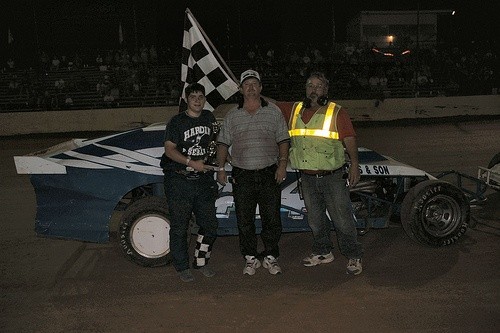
[{"xmin": 302, "ymin": 96, "xmax": 329, "ymax": 108}]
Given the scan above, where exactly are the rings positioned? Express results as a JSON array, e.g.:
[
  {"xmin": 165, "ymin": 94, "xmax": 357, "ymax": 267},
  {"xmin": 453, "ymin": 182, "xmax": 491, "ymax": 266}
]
[{"xmin": 283, "ymin": 178, "xmax": 285, "ymax": 180}]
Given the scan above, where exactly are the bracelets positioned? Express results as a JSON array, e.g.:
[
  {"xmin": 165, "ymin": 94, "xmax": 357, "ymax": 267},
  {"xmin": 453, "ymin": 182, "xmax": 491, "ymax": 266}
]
[
  {"xmin": 186, "ymin": 158, "xmax": 190, "ymax": 166},
  {"xmin": 280, "ymin": 158, "xmax": 287, "ymax": 161}
]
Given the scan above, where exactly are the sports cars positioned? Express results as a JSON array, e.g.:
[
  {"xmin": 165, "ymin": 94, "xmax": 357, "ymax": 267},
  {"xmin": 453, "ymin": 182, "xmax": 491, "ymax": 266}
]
[{"xmin": 12, "ymin": 118, "xmax": 491, "ymax": 267}]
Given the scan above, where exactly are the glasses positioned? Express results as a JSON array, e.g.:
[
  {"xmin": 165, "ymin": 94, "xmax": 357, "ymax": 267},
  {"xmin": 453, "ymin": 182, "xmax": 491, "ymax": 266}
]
[{"xmin": 305, "ymin": 82, "xmax": 326, "ymax": 91}]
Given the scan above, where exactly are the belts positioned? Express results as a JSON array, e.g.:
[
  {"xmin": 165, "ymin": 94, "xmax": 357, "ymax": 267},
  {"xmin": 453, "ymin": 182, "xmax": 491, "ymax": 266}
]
[{"xmin": 298, "ymin": 164, "xmax": 346, "ymax": 178}]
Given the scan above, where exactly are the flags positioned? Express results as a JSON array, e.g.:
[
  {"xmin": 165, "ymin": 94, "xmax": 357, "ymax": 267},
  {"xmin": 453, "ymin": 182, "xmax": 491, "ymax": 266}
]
[{"xmin": 179, "ymin": 12, "xmax": 241, "ymax": 112}]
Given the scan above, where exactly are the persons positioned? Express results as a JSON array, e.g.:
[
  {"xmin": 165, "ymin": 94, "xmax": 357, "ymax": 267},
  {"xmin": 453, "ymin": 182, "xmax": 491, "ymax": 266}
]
[
  {"xmin": 162, "ymin": 83, "xmax": 232, "ymax": 282},
  {"xmin": 216, "ymin": 70, "xmax": 291, "ymax": 276},
  {"xmin": 0, "ymin": 44, "xmax": 182, "ymax": 109},
  {"xmin": 249, "ymin": 42, "xmax": 500, "ymax": 97},
  {"xmin": 260, "ymin": 73, "xmax": 363, "ymax": 276}
]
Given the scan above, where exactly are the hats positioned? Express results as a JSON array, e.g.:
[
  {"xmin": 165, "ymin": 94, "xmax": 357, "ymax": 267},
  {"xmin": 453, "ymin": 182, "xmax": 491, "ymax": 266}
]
[{"xmin": 240, "ymin": 70, "xmax": 260, "ymax": 85}]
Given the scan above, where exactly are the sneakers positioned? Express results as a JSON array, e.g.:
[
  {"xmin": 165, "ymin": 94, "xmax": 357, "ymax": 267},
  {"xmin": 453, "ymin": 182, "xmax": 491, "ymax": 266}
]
[
  {"xmin": 176, "ymin": 269, "xmax": 195, "ymax": 282},
  {"xmin": 345, "ymin": 258, "xmax": 362, "ymax": 275},
  {"xmin": 242, "ymin": 255, "xmax": 261, "ymax": 275},
  {"xmin": 262, "ymin": 255, "xmax": 282, "ymax": 275},
  {"xmin": 302, "ymin": 252, "xmax": 335, "ymax": 267},
  {"xmin": 194, "ymin": 267, "xmax": 216, "ymax": 277}
]
